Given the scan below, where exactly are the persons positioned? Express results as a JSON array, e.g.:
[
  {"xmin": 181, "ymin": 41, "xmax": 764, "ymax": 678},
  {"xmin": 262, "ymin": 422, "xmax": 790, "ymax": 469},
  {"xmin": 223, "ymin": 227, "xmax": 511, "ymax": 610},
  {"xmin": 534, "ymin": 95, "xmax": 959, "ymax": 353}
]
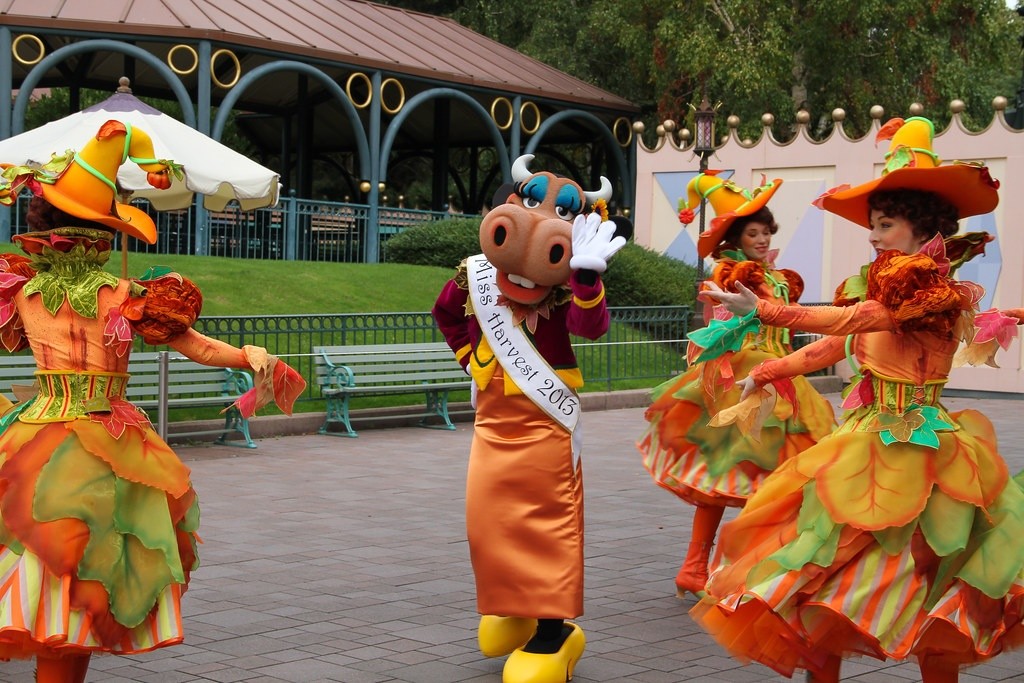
[
  {"xmin": 637, "ymin": 171, "xmax": 838, "ymax": 600},
  {"xmin": 690, "ymin": 116, "xmax": 1024, "ymax": 683},
  {"xmin": 0, "ymin": 120, "xmax": 307, "ymax": 683}
]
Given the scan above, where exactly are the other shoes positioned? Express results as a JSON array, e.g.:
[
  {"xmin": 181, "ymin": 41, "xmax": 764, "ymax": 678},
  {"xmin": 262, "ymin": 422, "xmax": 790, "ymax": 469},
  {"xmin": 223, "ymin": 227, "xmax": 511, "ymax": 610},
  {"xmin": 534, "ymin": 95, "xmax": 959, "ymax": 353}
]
[{"xmin": 477, "ymin": 612, "xmax": 585, "ymax": 683}]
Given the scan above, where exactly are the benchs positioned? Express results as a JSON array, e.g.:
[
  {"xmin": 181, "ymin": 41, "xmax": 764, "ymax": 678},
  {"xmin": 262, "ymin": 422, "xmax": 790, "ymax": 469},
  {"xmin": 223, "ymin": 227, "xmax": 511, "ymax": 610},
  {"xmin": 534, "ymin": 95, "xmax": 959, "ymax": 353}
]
[
  {"xmin": 313, "ymin": 342, "xmax": 475, "ymax": 439},
  {"xmin": 0, "ymin": 350, "xmax": 257, "ymax": 449}
]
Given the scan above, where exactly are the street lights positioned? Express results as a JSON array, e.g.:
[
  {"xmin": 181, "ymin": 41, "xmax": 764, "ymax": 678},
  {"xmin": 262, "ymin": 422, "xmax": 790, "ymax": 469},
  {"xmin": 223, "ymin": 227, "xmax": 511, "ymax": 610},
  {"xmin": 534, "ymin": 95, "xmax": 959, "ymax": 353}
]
[{"xmin": 686, "ymin": 93, "xmax": 723, "ymax": 332}]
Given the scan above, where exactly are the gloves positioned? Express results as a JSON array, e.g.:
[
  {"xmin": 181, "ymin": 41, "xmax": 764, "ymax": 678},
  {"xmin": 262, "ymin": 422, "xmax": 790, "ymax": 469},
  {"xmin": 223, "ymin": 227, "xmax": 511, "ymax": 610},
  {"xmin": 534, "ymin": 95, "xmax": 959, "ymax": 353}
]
[{"xmin": 569, "ymin": 212, "xmax": 627, "ymax": 275}]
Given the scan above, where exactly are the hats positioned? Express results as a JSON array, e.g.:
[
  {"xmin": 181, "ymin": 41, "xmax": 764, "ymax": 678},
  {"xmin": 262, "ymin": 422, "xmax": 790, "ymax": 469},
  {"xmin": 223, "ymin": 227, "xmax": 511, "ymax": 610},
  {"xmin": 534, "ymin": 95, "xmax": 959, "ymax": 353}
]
[
  {"xmin": 679, "ymin": 171, "xmax": 782, "ymax": 259},
  {"xmin": 0, "ymin": 120, "xmax": 180, "ymax": 246},
  {"xmin": 812, "ymin": 115, "xmax": 1000, "ymax": 230}
]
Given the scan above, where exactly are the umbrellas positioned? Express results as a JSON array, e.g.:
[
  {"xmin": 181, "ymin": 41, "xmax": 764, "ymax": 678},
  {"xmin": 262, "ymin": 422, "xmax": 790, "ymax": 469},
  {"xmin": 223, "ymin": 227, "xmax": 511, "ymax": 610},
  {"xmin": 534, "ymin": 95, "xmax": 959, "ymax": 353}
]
[{"xmin": 0, "ymin": 77, "xmax": 285, "ymax": 280}]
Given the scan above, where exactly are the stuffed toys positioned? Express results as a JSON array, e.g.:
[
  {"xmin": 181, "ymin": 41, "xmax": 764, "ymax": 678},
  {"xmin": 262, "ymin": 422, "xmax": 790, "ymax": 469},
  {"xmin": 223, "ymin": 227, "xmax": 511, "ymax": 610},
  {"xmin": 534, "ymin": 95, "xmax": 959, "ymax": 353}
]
[{"xmin": 431, "ymin": 154, "xmax": 626, "ymax": 683}]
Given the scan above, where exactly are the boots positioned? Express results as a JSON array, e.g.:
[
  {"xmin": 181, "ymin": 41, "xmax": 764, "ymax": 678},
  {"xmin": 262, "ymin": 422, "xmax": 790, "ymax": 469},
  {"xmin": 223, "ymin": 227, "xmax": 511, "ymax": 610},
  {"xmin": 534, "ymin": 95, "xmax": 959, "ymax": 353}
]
[{"xmin": 675, "ymin": 541, "xmax": 712, "ymax": 600}]
